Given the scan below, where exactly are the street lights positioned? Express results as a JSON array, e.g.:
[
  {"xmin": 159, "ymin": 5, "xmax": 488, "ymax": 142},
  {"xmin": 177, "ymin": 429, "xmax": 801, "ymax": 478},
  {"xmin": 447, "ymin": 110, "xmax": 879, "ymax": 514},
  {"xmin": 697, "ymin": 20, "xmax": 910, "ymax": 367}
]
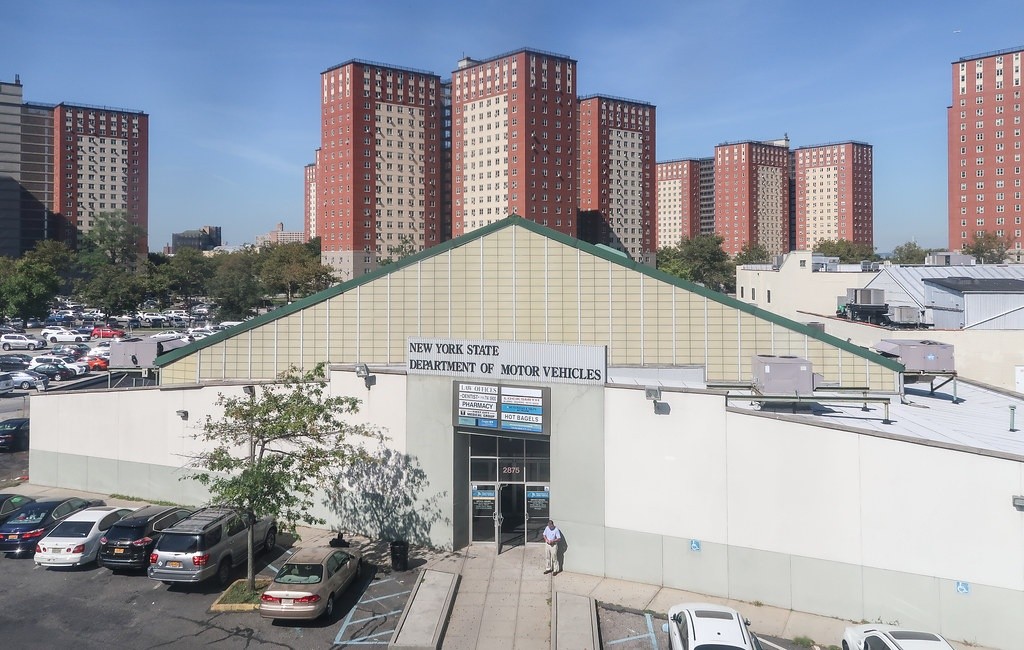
[{"xmin": 243, "ymin": 385, "xmax": 256, "ymax": 590}]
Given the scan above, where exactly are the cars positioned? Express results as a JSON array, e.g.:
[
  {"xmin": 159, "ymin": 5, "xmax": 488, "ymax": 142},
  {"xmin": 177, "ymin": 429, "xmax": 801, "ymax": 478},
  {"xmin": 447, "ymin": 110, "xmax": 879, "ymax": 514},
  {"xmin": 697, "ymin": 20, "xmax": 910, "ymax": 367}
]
[
  {"xmin": 259, "ymin": 545, "xmax": 363, "ymax": 622},
  {"xmin": 0, "ymin": 296, "xmax": 260, "ymax": 395},
  {"xmin": 47, "ymin": 329, "xmax": 91, "ymax": 342},
  {"xmin": 0, "ymin": 494, "xmax": 37, "ymax": 524},
  {"xmin": 33, "ymin": 505, "xmax": 139, "ymax": 568},
  {"xmin": 0, "ymin": 496, "xmax": 107, "ymax": 557},
  {"xmin": 0, "ymin": 418, "xmax": 29, "ymax": 452},
  {"xmin": 841, "ymin": 623, "xmax": 954, "ymax": 650},
  {"xmin": 91, "ymin": 327, "xmax": 124, "ymax": 339},
  {"xmin": 666, "ymin": 602, "xmax": 764, "ymax": 650}
]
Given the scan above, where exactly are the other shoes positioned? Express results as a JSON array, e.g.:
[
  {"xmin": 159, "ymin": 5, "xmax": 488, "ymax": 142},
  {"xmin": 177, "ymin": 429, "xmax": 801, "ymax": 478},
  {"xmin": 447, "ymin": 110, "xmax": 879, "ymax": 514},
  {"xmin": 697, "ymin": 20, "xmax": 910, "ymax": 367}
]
[
  {"xmin": 553, "ymin": 572, "xmax": 557, "ymax": 576},
  {"xmin": 544, "ymin": 570, "xmax": 552, "ymax": 574}
]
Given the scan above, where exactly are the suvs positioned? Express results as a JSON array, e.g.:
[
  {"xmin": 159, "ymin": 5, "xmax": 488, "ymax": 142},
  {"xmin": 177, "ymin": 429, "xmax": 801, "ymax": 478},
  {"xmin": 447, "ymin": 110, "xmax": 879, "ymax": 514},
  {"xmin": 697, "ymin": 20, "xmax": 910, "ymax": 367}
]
[
  {"xmin": 1, "ymin": 333, "xmax": 47, "ymax": 351},
  {"xmin": 147, "ymin": 504, "xmax": 277, "ymax": 587},
  {"xmin": 97, "ymin": 504, "xmax": 192, "ymax": 573}
]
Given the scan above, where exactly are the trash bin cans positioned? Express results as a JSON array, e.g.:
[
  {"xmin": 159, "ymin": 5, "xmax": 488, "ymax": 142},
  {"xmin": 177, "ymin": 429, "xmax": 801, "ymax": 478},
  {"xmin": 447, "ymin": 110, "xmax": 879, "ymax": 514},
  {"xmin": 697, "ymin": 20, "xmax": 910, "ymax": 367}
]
[{"xmin": 390, "ymin": 540, "xmax": 409, "ymax": 572}]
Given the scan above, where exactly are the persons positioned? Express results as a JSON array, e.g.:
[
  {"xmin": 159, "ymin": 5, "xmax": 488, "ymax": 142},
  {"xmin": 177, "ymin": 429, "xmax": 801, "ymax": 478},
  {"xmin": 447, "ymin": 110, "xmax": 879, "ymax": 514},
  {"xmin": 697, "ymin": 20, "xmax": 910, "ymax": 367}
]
[
  {"xmin": 329, "ymin": 532, "xmax": 351, "ymax": 548},
  {"xmin": 542, "ymin": 520, "xmax": 562, "ymax": 576}
]
